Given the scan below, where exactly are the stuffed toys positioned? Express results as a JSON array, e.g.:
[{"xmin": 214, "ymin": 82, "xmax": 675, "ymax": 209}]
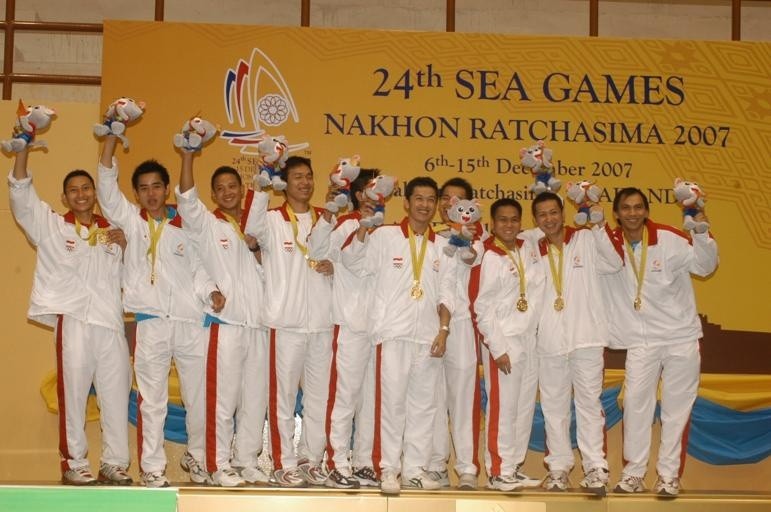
[
  {"xmin": 442, "ymin": 195, "xmax": 482, "ymax": 261},
  {"xmin": 254, "ymin": 133, "xmax": 291, "ymax": 192},
  {"xmin": 518, "ymin": 140, "xmax": 563, "ymax": 195},
  {"xmin": 1, "ymin": 100, "xmax": 57, "ymax": 154},
  {"xmin": 671, "ymin": 177, "xmax": 710, "ymax": 233},
  {"xmin": 326, "ymin": 152, "xmax": 361, "ymax": 212},
  {"xmin": 171, "ymin": 114, "xmax": 218, "ymax": 152},
  {"xmin": 567, "ymin": 179, "xmax": 604, "ymax": 226},
  {"xmin": 359, "ymin": 175, "xmax": 397, "ymax": 228},
  {"xmin": 90, "ymin": 93, "xmax": 148, "ymax": 151}
]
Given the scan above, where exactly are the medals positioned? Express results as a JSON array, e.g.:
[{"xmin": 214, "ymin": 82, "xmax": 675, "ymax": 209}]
[
  {"xmin": 633, "ymin": 297, "xmax": 643, "ymax": 311},
  {"xmin": 309, "ymin": 259, "xmax": 317, "ymax": 269},
  {"xmin": 554, "ymin": 297, "xmax": 564, "ymax": 310},
  {"xmin": 410, "ymin": 287, "xmax": 424, "ymax": 300},
  {"xmin": 150, "ymin": 273, "xmax": 156, "ymax": 286},
  {"xmin": 98, "ymin": 228, "xmax": 110, "ymax": 244},
  {"xmin": 516, "ymin": 298, "xmax": 528, "ymax": 312}
]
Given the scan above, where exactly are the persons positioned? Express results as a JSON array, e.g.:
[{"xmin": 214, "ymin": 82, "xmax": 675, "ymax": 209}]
[
  {"xmin": 93, "ymin": 131, "xmax": 227, "ymax": 489},
  {"xmin": 6, "ymin": 120, "xmax": 134, "ymax": 485},
  {"xmin": 466, "ymin": 195, "xmax": 546, "ymax": 491},
  {"xmin": 606, "ymin": 184, "xmax": 719, "ymax": 496},
  {"xmin": 171, "ymin": 143, "xmax": 270, "ymax": 487},
  {"xmin": 521, "ymin": 191, "xmax": 625, "ymax": 493},
  {"xmin": 338, "ymin": 174, "xmax": 459, "ymax": 496},
  {"xmin": 238, "ymin": 154, "xmax": 336, "ymax": 490},
  {"xmin": 428, "ymin": 176, "xmax": 493, "ymax": 494},
  {"xmin": 303, "ymin": 165, "xmax": 382, "ymax": 494}
]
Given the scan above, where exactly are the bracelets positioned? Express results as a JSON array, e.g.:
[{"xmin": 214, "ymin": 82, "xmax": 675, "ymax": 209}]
[
  {"xmin": 247, "ymin": 245, "xmax": 260, "ymax": 251},
  {"xmin": 440, "ymin": 325, "xmax": 449, "ymax": 334}
]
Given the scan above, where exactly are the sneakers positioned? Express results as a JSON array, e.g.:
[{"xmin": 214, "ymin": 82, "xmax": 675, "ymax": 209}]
[
  {"xmin": 178, "ymin": 451, "xmax": 211, "ymax": 485},
  {"xmin": 268, "ymin": 467, "xmax": 309, "ymax": 488},
  {"xmin": 457, "ymin": 474, "xmax": 477, "ymax": 491},
  {"xmin": 352, "ymin": 466, "xmax": 381, "ymax": 487},
  {"xmin": 380, "ymin": 468, "xmax": 403, "ymax": 495},
  {"xmin": 97, "ymin": 462, "xmax": 133, "ymax": 485},
  {"xmin": 139, "ymin": 471, "xmax": 171, "ymax": 488},
  {"xmin": 232, "ymin": 463, "xmax": 270, "ymax": 485},
  {"xmin": 578, "ymin": 467, "xmax": 610, "ymax": 497},
  {"xmin": 543, "ymin": 469, "xmax": 570, "ymax": 492},
  {"xmin": 325, "ymin": 468, "xmax": 361, "ymax": 490},
  {"xmin": 401, "ymin": 467, "xmax": 450, "ymax": 490},
  {"xmin": 212, "ymin": 467, "xmax": 246, "ymax": 487},
  {"xmin": 61, "ymin": 465, "xmax": 97, "ymax": 486},
  {"xmin": 612, "ymin": 473, "xmax": 646, "ymax": 493},
  {"xmin": 296, "ymin": 458, "xmax": 327, "ymax": 486},
  {"xmin": 486, "ymin": 469, "xmax": 542, "ymax": 492},
  {"xmin": 654, "ymin": 476, "xmax": 681, "ymax": 496}
]
